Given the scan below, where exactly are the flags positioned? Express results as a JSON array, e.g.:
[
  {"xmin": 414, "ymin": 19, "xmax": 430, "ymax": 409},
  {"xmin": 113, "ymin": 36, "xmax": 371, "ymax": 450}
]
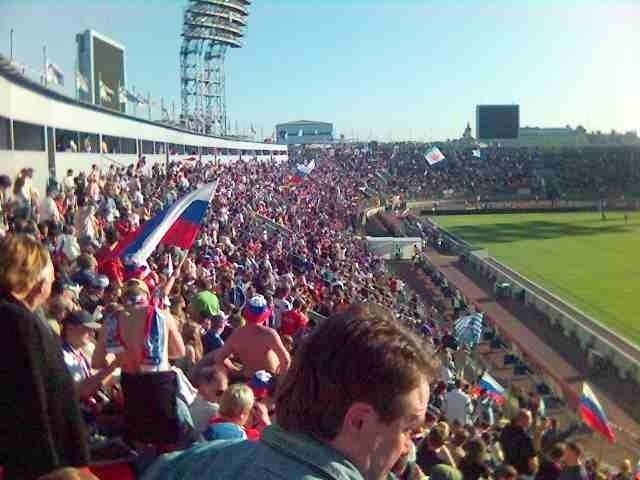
[
  {"xmin": 151, "ymin": 98, "xmax": 256, "ymax": 134},
  {"xmin": 99, "ymin": 80, "xmax": 113, "ymax": 103},
  {"xmin": 47, "ymin": 62, "xmax": 65, "ymax": 87},
  {"xmin": 74, "ymin": 71, "xmax": 90, "ymax": 92},
  {"xmin": 12, "ymin": 56, "xmax": 26, "ymax": 75},
  {"xmin": 134, "ymin": 91, "xmax": 149, "ymax": 105},
  {"xmin": 118, "ymin": 87, "xmax": 139, "ymax": 104}
]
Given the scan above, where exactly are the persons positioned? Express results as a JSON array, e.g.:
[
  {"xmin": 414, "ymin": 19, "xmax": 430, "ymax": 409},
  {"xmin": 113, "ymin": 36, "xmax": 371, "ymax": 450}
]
[{"xmin": 1, "ymin": 135, "xmax": 639, "ymax": 479}]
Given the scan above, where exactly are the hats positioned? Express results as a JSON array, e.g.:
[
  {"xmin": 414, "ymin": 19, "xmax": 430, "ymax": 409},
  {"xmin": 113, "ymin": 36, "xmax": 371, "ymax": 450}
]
[
  {"xmin": 121, "ymin": 278, "xmax": 150, "ymax": 301},
  {"xmin": 241, "ymin": 295, "xmax": 273, "ymax": 322},
  {"xmin": 64, "ymin": 309, "xmax": 102, "ymax": 329}
]
